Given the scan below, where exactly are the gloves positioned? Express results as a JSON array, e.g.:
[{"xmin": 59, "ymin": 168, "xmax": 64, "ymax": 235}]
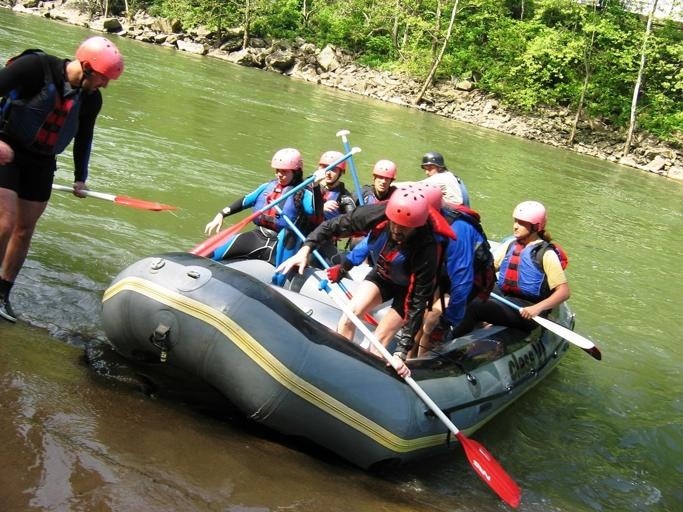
[{"xmin": 325, "ymin": 263, "xmax": 348, "ymax": 284}]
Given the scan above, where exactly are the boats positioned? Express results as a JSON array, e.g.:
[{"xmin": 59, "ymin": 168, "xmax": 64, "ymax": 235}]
[{"xmin": 92, "ymin": 235, "xmax": 576, "ymax": 471}]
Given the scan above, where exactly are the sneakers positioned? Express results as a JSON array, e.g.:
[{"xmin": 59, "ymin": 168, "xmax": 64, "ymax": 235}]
[{"xmin": 0, "ymin": 290, "xmax": 18, "ymax": 322}]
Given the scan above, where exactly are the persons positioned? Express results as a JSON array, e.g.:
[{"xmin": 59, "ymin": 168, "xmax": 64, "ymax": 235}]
[{"xmin": 2, "ymin": 36, "xmax": 123, "ymax": 324}]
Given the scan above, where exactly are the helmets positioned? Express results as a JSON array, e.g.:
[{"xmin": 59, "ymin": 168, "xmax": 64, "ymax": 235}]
[
  {"xmin": 372, "ymin": 159, "xmax": 398, "ymax": 179},
  {"xmin": 421, "ymin": 151, "xmax": 445, "ymax": 169},
  {"xmin": 511, "ymin": 200, "xmax": 547, "ymax": 233},
  {"xmin": 318, "ymin": 150, "xmax": 347, "ymax": 174},
  {"xmin": 384, "ymin": 183, "xmax": 443, "ymax": 228},
  {"xmin": 270, "ymin": 148, "xmax": 304, "ymax": 171},
  {"xmin": 75, "ymin": 35, "xmax": 124, "ymax": 81}
]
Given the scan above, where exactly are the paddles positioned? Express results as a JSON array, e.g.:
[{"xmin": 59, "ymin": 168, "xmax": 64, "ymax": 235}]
[
  {"xmin": 489, "ymin": 292, "xmax": 600, "ymax": 361},
  {"xmin": 187, "ymin": 147, "xmax": 362, "ymax": 259},
  {"xmin": 51, "ymin": 184, "xmax": 176, "ymax": 211},
  {"xmin": 315, "ymin": 278, "xmax": 523, "ymax": 509},
  {"xmin": 260, "ymin": 191, "xmax": 378, "ymax": 327}
]
